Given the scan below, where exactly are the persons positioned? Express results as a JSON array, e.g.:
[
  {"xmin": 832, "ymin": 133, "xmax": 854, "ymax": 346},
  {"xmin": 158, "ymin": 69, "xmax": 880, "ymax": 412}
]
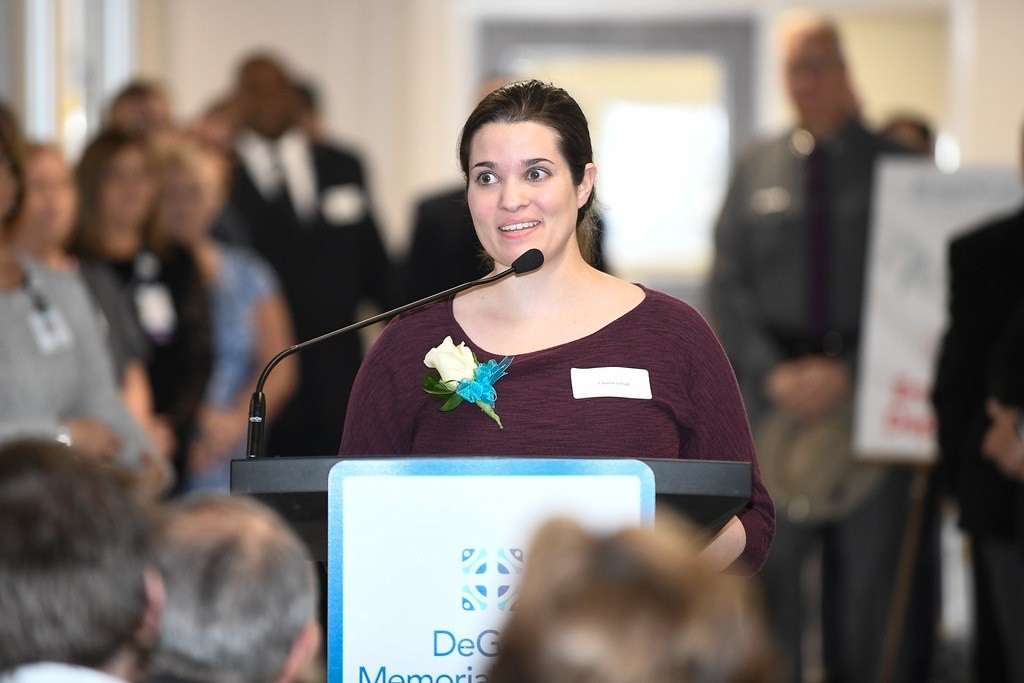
[
  {"xmin": 135, "ymin": 488, "xmax": 322, "ymax": 683},
  {"xmin": 0, "ymin": 436, "xmax": 166, "ymax": 683},
  {"xmin": 337, "ymin": 81, "xmax": 775, "ymax": 574},
  {"xmin": 2, "ymin": 49, "xmax": 608, "ymax": 516},
  {"xmin": 482, "ymin": 515, "xmax": 780, "ymax": 683},
  {"xmin": 709, "ymin": 15, "xmax": 1023, "ymax": 683}
]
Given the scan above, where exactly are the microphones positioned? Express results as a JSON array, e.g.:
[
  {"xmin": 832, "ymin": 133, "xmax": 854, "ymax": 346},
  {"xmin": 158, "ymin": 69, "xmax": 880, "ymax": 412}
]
[{"xmin": 245, "ymin": 248, "xmax": 545, "ymax": 459}]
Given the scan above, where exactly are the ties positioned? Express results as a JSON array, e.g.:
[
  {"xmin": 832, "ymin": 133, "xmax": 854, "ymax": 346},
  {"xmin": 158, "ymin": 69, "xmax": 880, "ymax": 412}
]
[{"xmin": 805, "ymin": 150, "xmax": 834, "ymax": 349}]
